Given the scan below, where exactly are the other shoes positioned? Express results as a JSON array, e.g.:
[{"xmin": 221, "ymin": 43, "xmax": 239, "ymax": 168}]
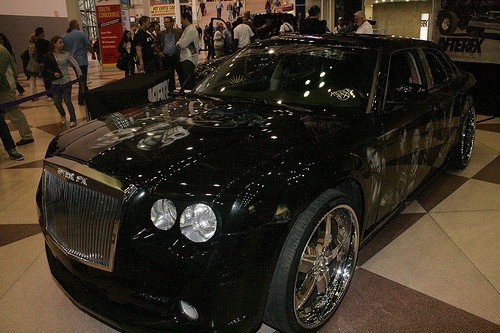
[
  {"xmin": 70, "ymin": 122, "xmax": 77, "ymax": 127},
  {"xmin": 8, "ymin": 148, "xmax": 24, "ymax": 161},
  {"xmin": 32, "ymin": 97, "xmax": 39, "ymax": 101},
  {"xmin": 19, "ymin": 90, "xmax": 26, "ymax": 96},
  {"xmin": 61, "ymin": 116, "xmax": 66, "ymax": 125},
  {"xmin": 16, "ymin": 138, "xmax": 34, "ymax": 146}
]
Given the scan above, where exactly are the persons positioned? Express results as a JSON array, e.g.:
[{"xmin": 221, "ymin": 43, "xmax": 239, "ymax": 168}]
[
  {"xmin": 44, "ymin": 36, "xmax": 83, "ymax": 127},
  {"xmin": 264, "ymin": 0, "xmax": 281, "ymax": 13},
  {"xmin": 195, "ymin": 24, "xmax": 202, "ymax": 52},
  {"xmin": 204, "ymin": 11, "xmax": 272, "ymax": 60},
  {"xmin": 200, "ymin": 1, "xmax": 205, "ymax": 16},
  {"xmin": 279, "ymin": 17, "xmax": 295, "ymax": 34},
  {"xmin": 117, "ymin": 16, "xmax": 160, "ymax": 77},
  {"xmin": 153, "ymin": 16, "xmax": 185, "ymax": 89},
  {"xmin": 176, "ymin": 11, "xmax": 200, "ymax": 92},
  {"xmin": 216, "ymin": 0, "xmax": 243, "ymax": 21},
  {"xmin": 25, "ymin": 27, "xmax": 55, "ymax": 101},
  {"xmin": 62, "ymin": 19, "xmax": 96, "ymax": 104},
  {"xmin": 300, "ymin": 5, "xmax": 358, "ymax": 35},
  {"xmin": 354, "ymin": 12, "xmax": 373, "ymax": 34},
  {"xmin": 94, "ymin": 37, "xmax": 102, "ymax": 65},
  {"xmin": 0, "ymin": 33, "xmax": 34, "ymax": 160}
]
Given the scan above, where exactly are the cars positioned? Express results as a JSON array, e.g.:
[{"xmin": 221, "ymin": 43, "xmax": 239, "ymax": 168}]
[
  {"xmin": 205, "ymin": 11, "xmax": 298, "ymax": 51},
  {"xmin": 34, "ymin": 29, "xmax": 479, "ymax": 333}
]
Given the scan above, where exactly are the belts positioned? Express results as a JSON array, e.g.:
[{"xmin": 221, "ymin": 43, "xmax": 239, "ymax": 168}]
[{"xmin": 164, "ymin": 54, "xmax": 178, "ymax": 57}]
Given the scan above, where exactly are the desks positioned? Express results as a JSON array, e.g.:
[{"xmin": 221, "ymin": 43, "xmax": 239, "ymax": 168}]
[{"xmin": 83, "ymin": 70, "xmax": 173, "ymax": 124}]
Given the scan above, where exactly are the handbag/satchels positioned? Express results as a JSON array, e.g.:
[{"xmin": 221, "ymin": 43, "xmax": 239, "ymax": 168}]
[
  {"xmin": 115, "ymin": 54, "xmax": 127, "ymax": 71},
  {"xmin": 32, "ymin": 48, "xmax": 38, "ymax": 62}
]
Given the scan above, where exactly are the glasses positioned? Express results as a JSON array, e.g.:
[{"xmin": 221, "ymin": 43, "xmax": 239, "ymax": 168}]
[{"xmin": 163, "ymin": 21, "xmax": 170, "ymax": 24}]
